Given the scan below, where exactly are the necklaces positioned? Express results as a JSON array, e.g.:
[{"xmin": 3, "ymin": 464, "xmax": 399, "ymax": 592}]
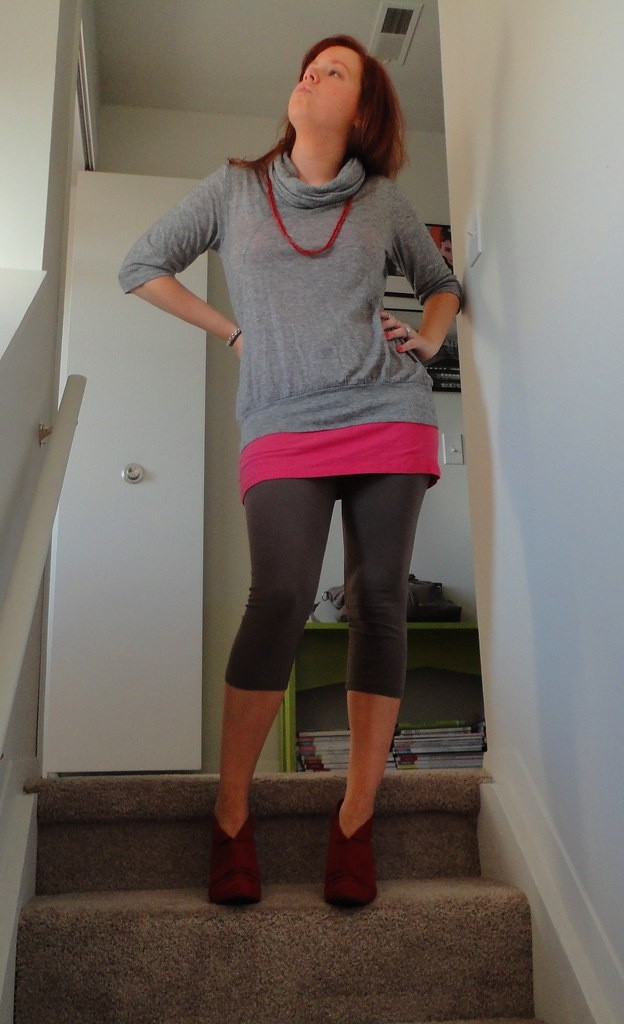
[{"xmin": 264, "ymin": 163, "xmax": 356, "ymax": 260}]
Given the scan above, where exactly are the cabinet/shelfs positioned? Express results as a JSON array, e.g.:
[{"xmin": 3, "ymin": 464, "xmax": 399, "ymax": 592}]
[
  {"xmin": 291, "ymin": 616, "xmax": 483, "ymax": 769},
  {"xmin": 45, "ymin": 165, "xmax": 299, "ymax": 772}
]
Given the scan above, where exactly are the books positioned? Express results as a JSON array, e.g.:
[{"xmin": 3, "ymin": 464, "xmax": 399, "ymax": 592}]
[{"xmin": 294, "ymin": 712, "xmax": 489, "ymax": 774}]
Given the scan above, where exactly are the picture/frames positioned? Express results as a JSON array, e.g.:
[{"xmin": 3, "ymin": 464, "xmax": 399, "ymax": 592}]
[
  {"xmin": 382, "ymin": 308, "xmax": 461, "ymax": 392},
  {"xmin": 383, "ymin": 222, "xmax": 452, "ymax": 299}
]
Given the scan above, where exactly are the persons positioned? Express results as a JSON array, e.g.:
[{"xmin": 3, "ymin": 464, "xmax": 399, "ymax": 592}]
[
  {"xmin": 117, "ymin": 32, "xmax": 468, "ymax": 909},
  {"xmin": 438, "ymin": 225, "xmax": 454, "ymax": 272}
]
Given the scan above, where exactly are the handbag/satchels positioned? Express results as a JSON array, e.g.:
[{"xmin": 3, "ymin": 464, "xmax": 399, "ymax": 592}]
[{"xmin": 322, "ymin": 575, "xmax": 462, "ymax": 624}]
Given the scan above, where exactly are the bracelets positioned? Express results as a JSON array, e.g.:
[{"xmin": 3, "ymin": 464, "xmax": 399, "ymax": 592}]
[{"xmin": 227, "ymin": 324, "xmax": 243, "ymax": 348}]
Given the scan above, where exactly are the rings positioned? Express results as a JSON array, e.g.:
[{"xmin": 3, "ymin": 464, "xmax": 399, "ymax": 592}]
[
  {"xmin": 386, "ymin": 311, "xmax": 392, "ymax": 321},
  {"xmin": 406, "ymin": 326, "xmax": 410, "ymax": 339}
]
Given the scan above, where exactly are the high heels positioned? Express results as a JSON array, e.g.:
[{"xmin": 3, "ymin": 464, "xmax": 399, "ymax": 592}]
[{"xmin": 208, "ymin": 799, "xmax": 379, "ymax": 910}]
